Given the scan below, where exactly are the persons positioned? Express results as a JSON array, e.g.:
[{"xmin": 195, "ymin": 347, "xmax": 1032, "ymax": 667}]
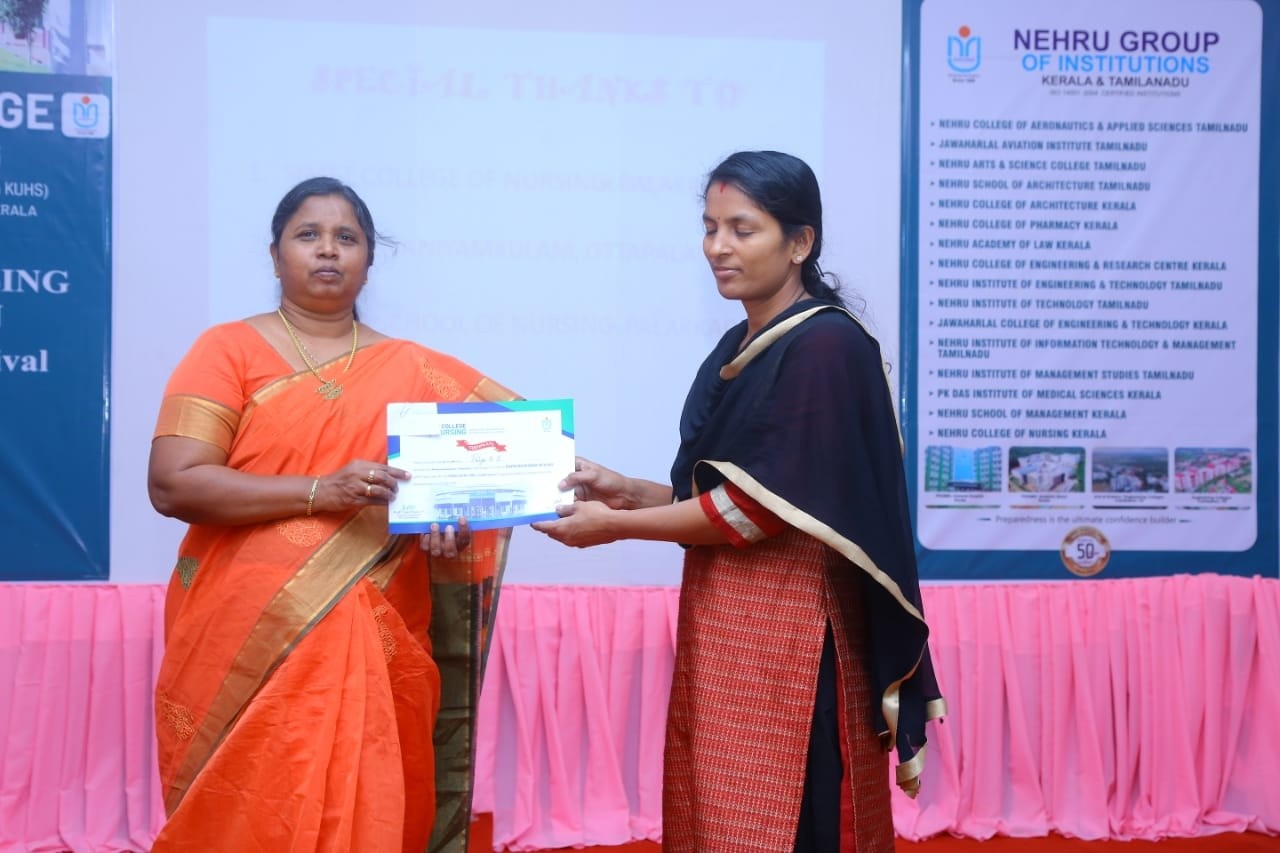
[
  {"xmin": 531, "ymin": 150, "xmax": 947, "ymax": 853},
  {"xmin": 147, "ymin": 178, "xmax": 535, "ymax": 853}
]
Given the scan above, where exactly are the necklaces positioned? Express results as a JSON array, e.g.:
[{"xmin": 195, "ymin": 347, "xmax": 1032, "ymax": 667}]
[{"xmin": 277, "ymin": 305, "xmax": 358, "ymax": 383}]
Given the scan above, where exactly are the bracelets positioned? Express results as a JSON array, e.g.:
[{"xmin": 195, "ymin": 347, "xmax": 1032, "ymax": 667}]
[{"xmin": 307, "ymin": 475, "xmax": 320, "ymax": 515}]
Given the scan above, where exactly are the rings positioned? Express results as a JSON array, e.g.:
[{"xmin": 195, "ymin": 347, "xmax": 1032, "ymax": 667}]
[
  {"xmin": 368, "ymin": 469, "xmax": 376, "ymax": 483},
  {"xmin": 366, "ymin": 485, "xmax": 372, "ymax": 497}
]
